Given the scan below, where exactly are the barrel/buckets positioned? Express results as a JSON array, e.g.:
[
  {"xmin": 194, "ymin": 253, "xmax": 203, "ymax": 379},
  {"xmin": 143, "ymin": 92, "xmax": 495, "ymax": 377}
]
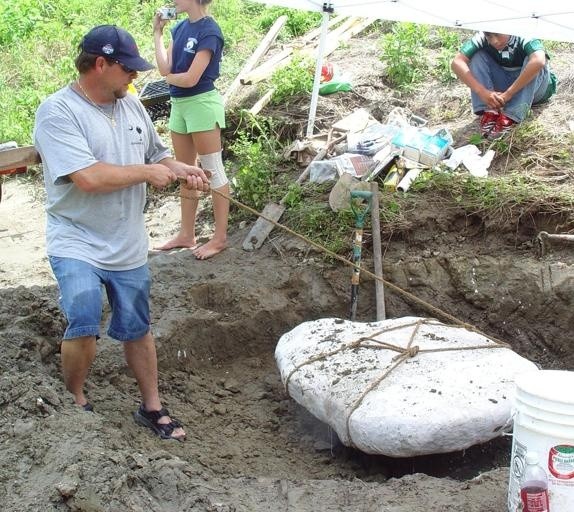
[{"xmin": 507, "ymin": 368, "xmax": 573, "ymax": 512}]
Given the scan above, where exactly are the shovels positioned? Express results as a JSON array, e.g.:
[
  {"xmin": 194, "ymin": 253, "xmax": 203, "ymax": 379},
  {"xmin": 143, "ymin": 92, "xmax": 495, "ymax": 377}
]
[{"xmin": 241, "ymin": 145, "xmax": 328, "ymax": 251}]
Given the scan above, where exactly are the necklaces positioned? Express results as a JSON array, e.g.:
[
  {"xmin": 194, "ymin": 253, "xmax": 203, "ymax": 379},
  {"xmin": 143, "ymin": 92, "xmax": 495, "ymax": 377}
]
[{"xmin": 76, "ymin": 76, "xmax": 117, "ymax": 126}]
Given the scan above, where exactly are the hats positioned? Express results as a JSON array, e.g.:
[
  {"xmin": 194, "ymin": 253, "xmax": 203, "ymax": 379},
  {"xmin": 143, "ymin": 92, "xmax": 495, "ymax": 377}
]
[{"xmin": 79, "ymin": 24, "xmax": 157, "ymax": 72}]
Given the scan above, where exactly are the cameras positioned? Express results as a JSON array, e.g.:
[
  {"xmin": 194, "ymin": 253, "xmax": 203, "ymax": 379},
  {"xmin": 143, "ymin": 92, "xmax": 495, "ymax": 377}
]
[{"xmin": 160, "ymin": 7, "xmax": 177, "ymax": 21}]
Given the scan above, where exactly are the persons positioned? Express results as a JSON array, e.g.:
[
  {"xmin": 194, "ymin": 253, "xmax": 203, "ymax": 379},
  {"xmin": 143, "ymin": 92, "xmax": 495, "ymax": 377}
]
[
  {"xmin": 150, "ymin": 0, "xmax": 232, "ymax": 260},
  {"xmin": 31, "ymin": 24, "xmax": 212, "ymax": 441},
  {"xmin": 452, "ymin": 30, "xmax": 557, "ymax": 142}
]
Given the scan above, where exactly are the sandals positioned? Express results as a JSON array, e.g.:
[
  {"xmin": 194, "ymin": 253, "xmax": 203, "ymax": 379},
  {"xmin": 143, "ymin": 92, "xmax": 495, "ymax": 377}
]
[{"xmin": 134, "ymin": 399, "xmax": 187, "ymax": 442}]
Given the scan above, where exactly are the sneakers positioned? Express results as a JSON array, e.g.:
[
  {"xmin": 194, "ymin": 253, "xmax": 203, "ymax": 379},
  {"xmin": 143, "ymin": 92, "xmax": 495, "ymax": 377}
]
[{"xmin": 477, "ymin": 109, "xmax": 515, "ymax": 144}]
